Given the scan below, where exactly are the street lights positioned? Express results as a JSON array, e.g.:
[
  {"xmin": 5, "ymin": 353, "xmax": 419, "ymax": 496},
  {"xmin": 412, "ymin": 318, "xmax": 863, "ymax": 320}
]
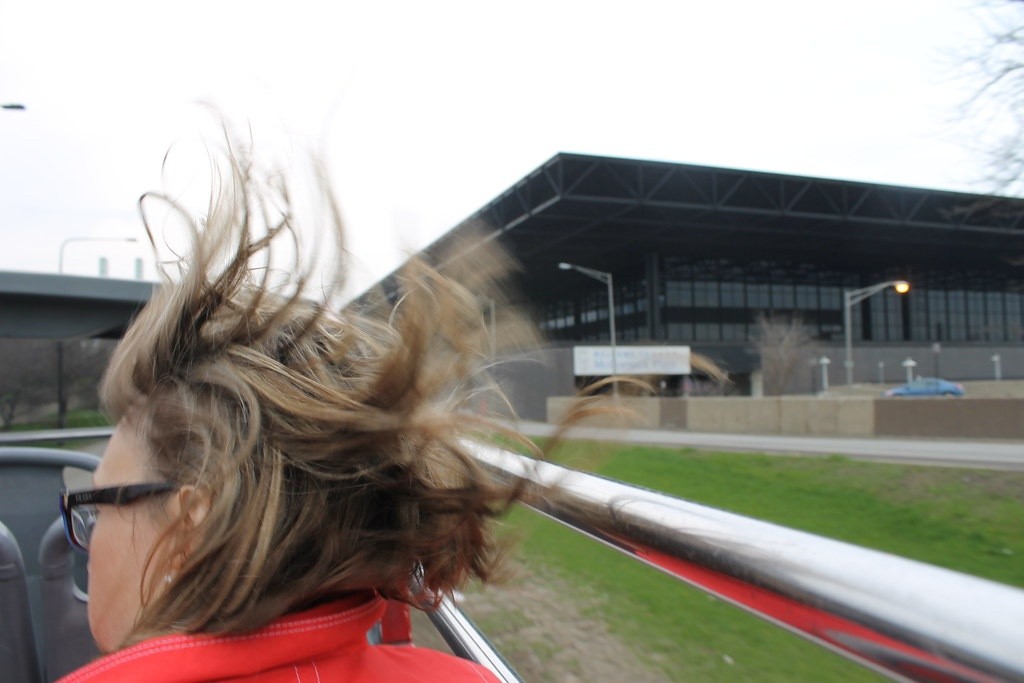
[
  {"xmin": 57, "ymin": 234, "xmax": 138, "ymax": 428},
  {"xmin": 843, "ymin": 280, "xmax": 911, "ymax": 386},
  {"xmin": 557, "ymin": 261, "xmax": 619, "ymax": 397}
]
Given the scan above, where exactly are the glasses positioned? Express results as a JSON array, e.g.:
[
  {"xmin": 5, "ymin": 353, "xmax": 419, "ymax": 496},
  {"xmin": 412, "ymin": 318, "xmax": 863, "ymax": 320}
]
[{"xmin": 60, "ymin": 481, "xmax": 180, "ymax": 554}]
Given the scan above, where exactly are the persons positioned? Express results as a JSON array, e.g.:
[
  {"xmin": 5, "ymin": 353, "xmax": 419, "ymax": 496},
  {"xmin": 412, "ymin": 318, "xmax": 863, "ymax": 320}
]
[{"xmin": 45, "ymin": 128, "xmax": 657, "ymax": 683}]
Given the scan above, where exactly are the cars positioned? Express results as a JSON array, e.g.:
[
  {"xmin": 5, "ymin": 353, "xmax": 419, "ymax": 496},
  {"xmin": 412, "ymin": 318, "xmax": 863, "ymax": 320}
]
[{"xmin": 881, "ymin": 378, "xmax": 963, "ymax": 398}]
